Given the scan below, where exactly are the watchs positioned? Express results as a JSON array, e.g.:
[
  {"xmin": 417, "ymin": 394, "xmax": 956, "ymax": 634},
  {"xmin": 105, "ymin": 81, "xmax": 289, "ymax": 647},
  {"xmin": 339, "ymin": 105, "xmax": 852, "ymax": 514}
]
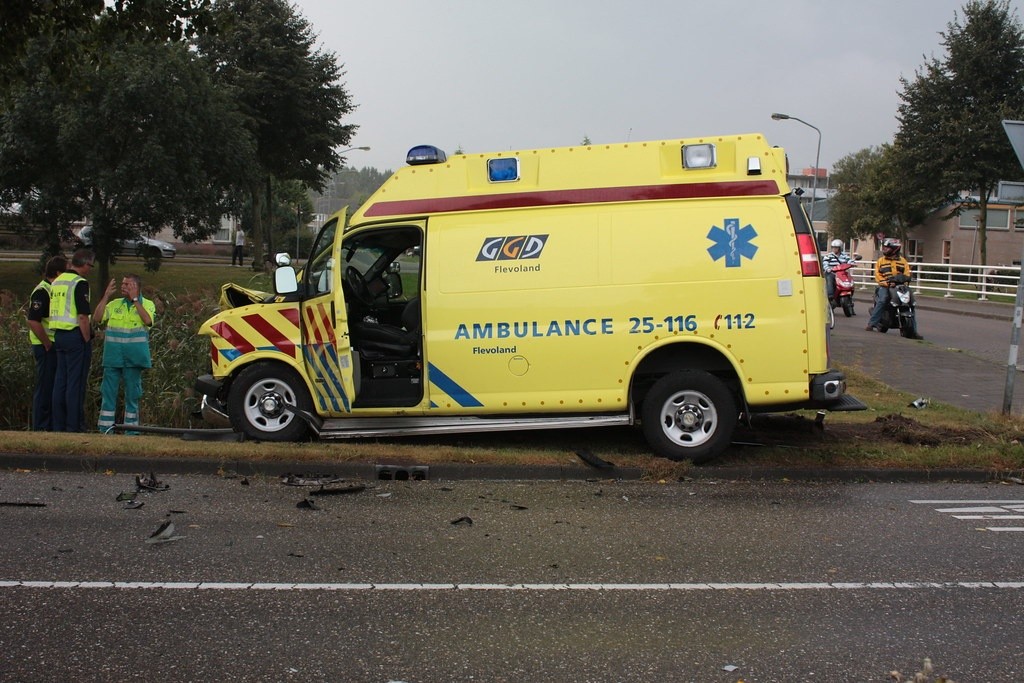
[{"xmin": 132, "ymin": 296, "xmax": 139, "ymax": 302}]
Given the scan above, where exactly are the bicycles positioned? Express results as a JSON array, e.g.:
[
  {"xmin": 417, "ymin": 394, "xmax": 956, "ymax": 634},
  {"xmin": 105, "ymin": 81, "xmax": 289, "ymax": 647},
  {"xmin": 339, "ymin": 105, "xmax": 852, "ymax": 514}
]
[{"xmin": 827, "ymin": 298, "xmax": 835, "ymax": 330}]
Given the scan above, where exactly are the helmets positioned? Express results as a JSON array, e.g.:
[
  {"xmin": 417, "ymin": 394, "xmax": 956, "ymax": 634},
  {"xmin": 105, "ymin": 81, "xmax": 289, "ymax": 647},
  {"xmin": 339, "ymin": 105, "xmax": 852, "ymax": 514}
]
[
  {"xmin": 882, "ymin": 239, "xmax": 901, "ymax": 257},
  {"xmin": 830, "ymin": 239, "xmax": 843, "ymax": 251}
]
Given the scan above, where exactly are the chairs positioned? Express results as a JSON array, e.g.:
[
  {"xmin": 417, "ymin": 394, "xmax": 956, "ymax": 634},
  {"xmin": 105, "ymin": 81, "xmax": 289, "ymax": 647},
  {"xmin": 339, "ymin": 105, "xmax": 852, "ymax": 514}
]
[{"xmin": 348, "ymin": 296, "xmax": 422, "ymax": 360}]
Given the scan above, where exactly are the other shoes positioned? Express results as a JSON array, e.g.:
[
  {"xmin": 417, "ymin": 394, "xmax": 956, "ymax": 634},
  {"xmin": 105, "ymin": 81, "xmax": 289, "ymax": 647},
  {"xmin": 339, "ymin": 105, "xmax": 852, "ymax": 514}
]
[
  {"xmin": 912, "ymin": 334, "xmax": 923, "ymax": 339},
  {"xmin": 866, "ymin": 326, "xmax": 873, "ymax": 331},
  {"xmin": 228, "ymin": 264, "xmax": 235, "ymax": 266},
  {"xmin": 852, "ymin": 307, "xmax": 856, "ymax": 315},
  {"xmin": 828, "ymin": 295, "xmax": 834, "ymax": 301},
  {"xmin": 235, "ymin": 264, "xmax": 243, "ymax": 267}
]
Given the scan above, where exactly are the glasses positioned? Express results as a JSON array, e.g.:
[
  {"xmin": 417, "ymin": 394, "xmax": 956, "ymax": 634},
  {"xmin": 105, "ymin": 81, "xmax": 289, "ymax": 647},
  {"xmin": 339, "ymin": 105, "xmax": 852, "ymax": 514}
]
[{"xmin": 86, "ymin": 261, "xmax": 95, "ymax": 268}]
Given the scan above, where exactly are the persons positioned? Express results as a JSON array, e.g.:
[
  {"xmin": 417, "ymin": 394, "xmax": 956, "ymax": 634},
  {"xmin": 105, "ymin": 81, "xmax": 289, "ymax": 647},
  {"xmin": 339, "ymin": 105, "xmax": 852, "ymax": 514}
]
[
  {"xmin": 865, "ymin": 238, "xmax": 910, "ymax": 330},
  {"xmin": 47, "ymin": 248, "xmax": 94, "ymax": 434},
  {"xmin": 228, "ymin": 224, "xmax": 245, "ymax": 267},
  {"xmin": 822, "ymin": 238, "xmax": 853, "ymax": 304},
  {"xmin": 27, "ymin": 256, "xmax": 71, "ymax": 434},
  {"xmin": 94, "ymin": 273, "xmax": 157, "ymax": 435}
]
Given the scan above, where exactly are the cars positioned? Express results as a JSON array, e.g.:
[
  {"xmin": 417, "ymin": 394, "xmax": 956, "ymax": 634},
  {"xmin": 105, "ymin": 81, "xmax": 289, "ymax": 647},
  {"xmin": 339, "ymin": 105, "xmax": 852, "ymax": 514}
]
[{"xmin": 73, "ymin": 223, "xmax": 176, "ymax": 259}]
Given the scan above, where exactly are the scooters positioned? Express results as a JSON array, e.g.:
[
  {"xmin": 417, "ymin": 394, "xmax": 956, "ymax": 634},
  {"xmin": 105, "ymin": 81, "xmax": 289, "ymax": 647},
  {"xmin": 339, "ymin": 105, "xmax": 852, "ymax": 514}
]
[
  {"xmin": 822, "ymin": 254, "xmax": 863, "ymax": 317},
  {"xmin": 868, "ymin": 267, "xmax": 915, "ymax": 339}
]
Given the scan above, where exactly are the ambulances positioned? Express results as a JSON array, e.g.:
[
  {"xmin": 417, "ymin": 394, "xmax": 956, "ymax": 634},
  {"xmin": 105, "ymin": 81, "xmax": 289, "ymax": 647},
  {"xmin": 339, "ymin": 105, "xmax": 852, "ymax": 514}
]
[{"xmin": 197, "ymin": 133, "xmax": 870, "ymax": 462}]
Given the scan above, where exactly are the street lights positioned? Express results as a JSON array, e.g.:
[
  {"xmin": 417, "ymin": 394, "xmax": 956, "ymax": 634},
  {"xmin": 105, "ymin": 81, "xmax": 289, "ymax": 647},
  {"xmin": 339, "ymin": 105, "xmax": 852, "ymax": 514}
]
[
  {"xmin": 316, "ymin": 146, "xmax": 371, "ymax": 249},
  {"xmin": 966, "ymin": 215, "xmax": 980, "ymax": 285},
  {"xmin": 770, "ymin": 113, "xmax": 821, "ymax": 222}
]
